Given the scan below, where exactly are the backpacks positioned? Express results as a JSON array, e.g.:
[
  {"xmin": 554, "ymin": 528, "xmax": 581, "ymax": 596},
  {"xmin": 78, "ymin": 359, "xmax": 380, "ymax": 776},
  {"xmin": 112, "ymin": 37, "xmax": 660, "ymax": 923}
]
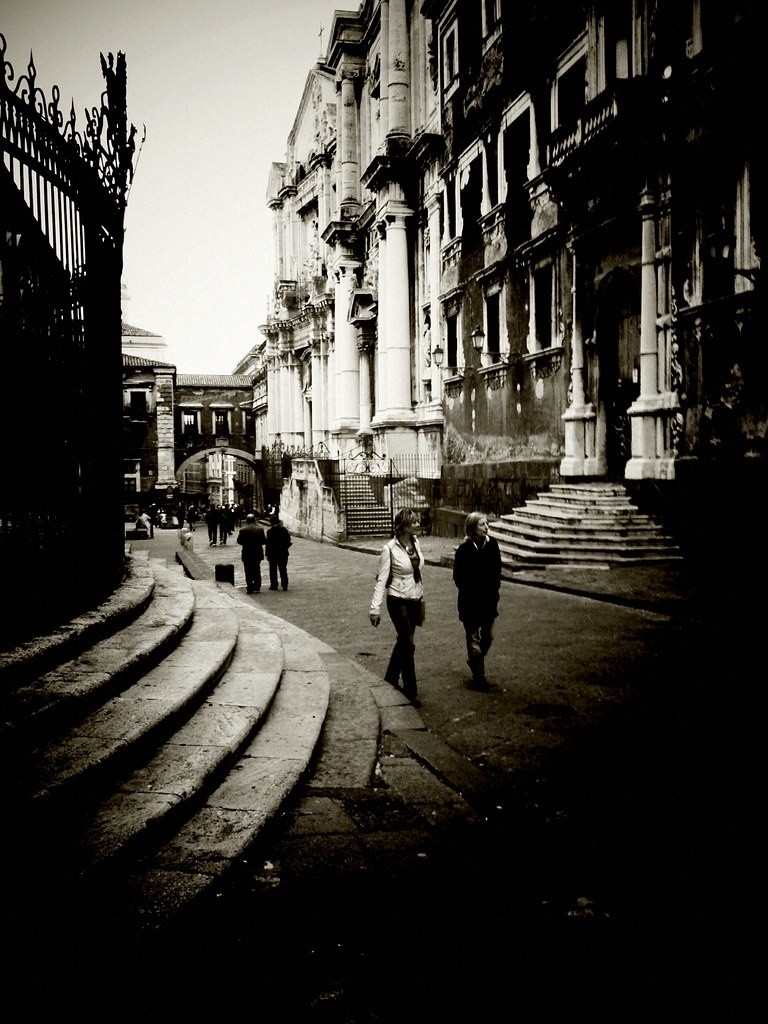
[{"xmin": 135, "ymin": 518, "xmax": 148, "ymax": 529}]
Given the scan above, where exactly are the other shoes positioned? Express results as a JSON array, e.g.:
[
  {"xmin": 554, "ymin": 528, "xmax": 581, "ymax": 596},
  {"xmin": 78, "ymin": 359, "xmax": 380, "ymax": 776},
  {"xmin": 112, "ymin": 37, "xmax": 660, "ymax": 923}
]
[
  {"xmin": 466, "ymin": 651, "xmax": 486, "ymax": 683},
  {"xmin": 269, "ymin": 586, "xmax": 278, "ymax": 590},
  {"xmin": 220, "ymin": 541, "xmax": 224, "ymax": 545},
  {"xmin": 229, "ymin": 533, "xmax": 233, "ymax": 535},
  {"xmin": 223, "ymin": 544, "xmax": 226, "ymax": 546},
  {"xmin": 385, "ymin": 677, "xmax": 403, "ymax": 692},
  {"xmin": 210, "ymin": 540, "xmax": 214, "ymax": 546},
  {"xmin": 282, "ymin": 584, "xmax": 288, "ymax": 591},
  {"xmin": 407, "ymin": 697, "xmax": 422, "ymax": 708},
  {"xmin": 212, "ymin": 544, "xmax": 217, "ymax": 547}
]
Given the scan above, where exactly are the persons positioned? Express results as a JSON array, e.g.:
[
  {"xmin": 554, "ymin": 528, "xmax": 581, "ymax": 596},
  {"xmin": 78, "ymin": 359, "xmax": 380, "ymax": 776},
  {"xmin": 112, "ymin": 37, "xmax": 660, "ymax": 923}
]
[
  {"xmin": 453, "ymin": 512, "xmax": 502, "ymax": 690},
  {"xmin": 369, "ymin": 508, "xmax": 426, "ymax": 707},
  {"xmin": 237, "ymin": 513, "xmax": 267, "ymax": 594},
  {"xmin": 265, "ymin": 515, "xmax": 293, "ymax": 591},
  {"xmin": 136, "ymin": 501, "xmax": 279, "ymax": 547}
]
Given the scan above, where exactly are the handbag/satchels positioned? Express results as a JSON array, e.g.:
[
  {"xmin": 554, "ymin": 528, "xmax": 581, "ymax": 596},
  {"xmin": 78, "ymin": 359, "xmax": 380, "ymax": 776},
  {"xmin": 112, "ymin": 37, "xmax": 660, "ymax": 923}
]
[
  {"xmin": 415, "ymin": 598, "xmax": 425, "ymax": 626},
  {"xmin": 375, "ymin": 544, "xmax": 393, "ymax": 589}
]
